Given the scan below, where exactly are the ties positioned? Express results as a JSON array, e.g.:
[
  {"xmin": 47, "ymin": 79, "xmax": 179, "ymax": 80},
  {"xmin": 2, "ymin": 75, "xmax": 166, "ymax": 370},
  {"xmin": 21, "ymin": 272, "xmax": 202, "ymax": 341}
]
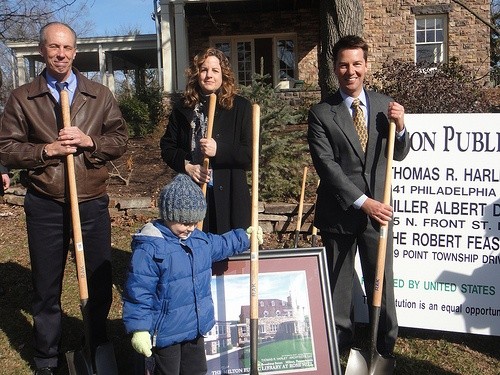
[
  {"xmin": 55, "ymin": 82, "xmax": 67, "ymax": 96},
  {"xmin": 351, "ymin": 98, "xmax": 368, "ymax": 155}
]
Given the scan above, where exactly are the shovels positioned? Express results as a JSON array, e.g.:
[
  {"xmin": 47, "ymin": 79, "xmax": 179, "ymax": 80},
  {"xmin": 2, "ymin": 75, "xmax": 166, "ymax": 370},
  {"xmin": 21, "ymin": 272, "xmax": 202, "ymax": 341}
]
[
  {"xmin": 294, "ymin": 167, "xmax": 308, "ymax": 248},
  {"xmin": 188, "ymin": 94, "xmax": 218, "ymax": 231},
  {"xmin": 312, "ymin": 180, "xmax": 322, "ymax": 247},
  {"xmin": 344, "ymin": 115, "xmax": 395, "ymax": 375},
  {"xmin": 57, "ymin": 90, "xmax": 118, "ymax": 375},
  {"xmin": 247, "ymin": 105, "xmax": 265, "ymax": 375}
]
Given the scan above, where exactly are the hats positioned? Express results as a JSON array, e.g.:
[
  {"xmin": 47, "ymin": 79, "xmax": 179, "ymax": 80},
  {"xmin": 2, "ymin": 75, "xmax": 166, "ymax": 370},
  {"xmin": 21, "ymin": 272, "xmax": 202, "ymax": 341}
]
[{"xmin": 159, "ymin": 173, "xmax": 207, "ymax": 224}]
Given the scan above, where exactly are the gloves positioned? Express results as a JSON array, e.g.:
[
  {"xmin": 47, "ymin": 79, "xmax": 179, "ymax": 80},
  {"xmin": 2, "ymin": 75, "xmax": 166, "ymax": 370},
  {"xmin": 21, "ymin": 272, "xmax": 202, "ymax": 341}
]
[
  {"xmin": 246, "ymin": 226, "xmax": 263, "ymax": 244},
  {"xmin": 131, "ymin": 331, "xmax": 152, "ymax": 357}
]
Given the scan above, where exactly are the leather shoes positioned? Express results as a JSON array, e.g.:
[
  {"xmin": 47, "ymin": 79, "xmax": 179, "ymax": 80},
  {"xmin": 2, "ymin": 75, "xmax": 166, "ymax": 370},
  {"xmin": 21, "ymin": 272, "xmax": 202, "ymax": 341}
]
[
  {"xmin": 35, "ymin": 367, "xmax": 53, "ymax": 375},
  {"xmin": 380, "ymin": 351, "xmax": 396, "ymax": 359},
  {"xmin": 80, "ymin": 336, "xmax": 111, "ymax": 345},
  {"xmin": 336, "ymin": 330, "xmax": 360, "ymax": 352}
]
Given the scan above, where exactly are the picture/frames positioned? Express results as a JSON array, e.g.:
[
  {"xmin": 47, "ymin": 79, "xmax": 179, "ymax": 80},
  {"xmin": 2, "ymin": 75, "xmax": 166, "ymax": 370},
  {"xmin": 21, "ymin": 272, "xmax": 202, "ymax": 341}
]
[{"xmin": 203, "ymin": 247, "xmax": 341, "ymax": 375}]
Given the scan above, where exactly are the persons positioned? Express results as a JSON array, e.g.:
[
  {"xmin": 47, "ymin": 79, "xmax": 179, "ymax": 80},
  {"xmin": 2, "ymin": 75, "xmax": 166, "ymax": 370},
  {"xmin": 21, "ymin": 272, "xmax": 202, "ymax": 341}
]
[
  {"xmin": 1, "ymin": 22, "xmax": 129, "ymax": 375},
  {"xmin": 308, "ymin": 34, "xmax": 411, "ymax": 361},
  {"xmin": 122, "ymin": 173, "xmax": 265, "ymax": 375},
  {"xmin": 160, "ymin": 49, "xmax": 263, "ymax": 253}
]
